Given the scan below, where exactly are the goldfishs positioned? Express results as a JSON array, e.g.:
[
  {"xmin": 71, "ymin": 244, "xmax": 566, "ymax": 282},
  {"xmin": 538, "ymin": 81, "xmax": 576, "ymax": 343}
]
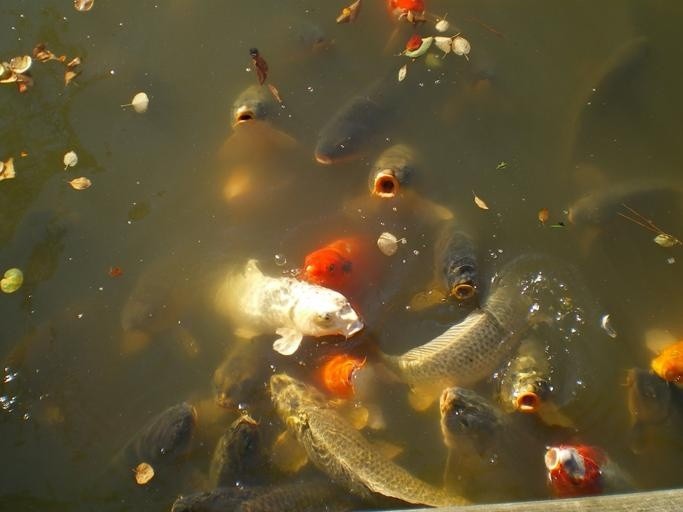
[{"xmin": 0, "ymin": 0, "xmax": 683, "ymax": 512}]
